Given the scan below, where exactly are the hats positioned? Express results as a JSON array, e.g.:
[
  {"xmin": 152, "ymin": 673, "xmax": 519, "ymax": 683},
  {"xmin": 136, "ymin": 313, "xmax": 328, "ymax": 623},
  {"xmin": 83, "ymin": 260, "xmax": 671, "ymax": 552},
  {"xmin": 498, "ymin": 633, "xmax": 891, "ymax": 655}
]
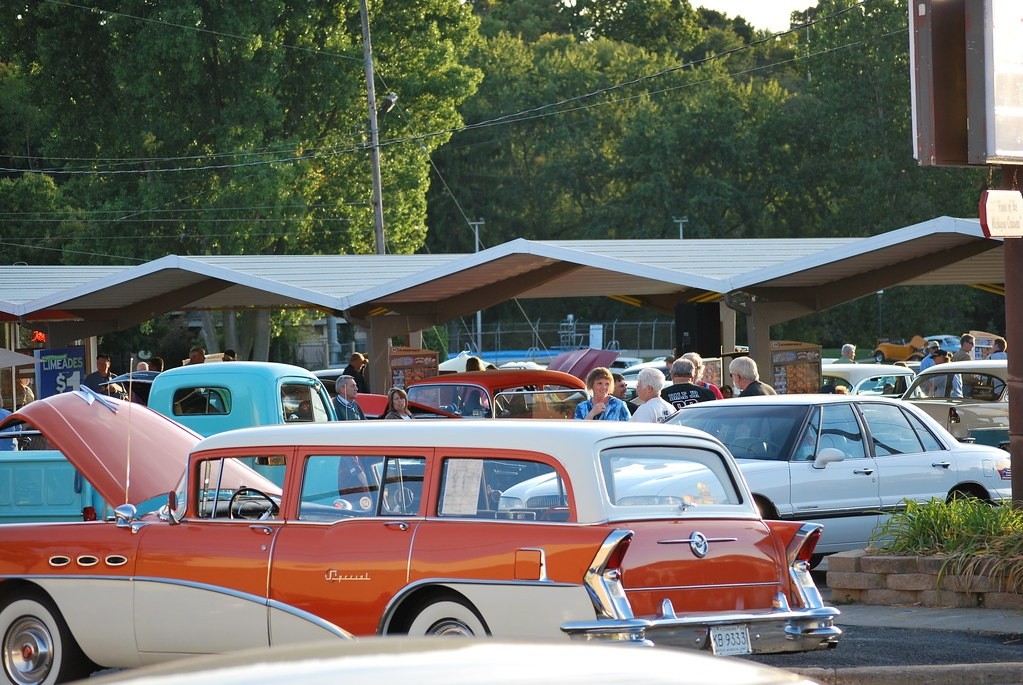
[
  {"xmin": 929, "ymin": 348, "xmax": 946, "ymax": 358},
  {"xmin": 928, "ymin": 340, "xmax": 939, "ymax": 347},
  {"xmin": 664, "ymin": 355, "xmax": 676, "ymax": 362}
]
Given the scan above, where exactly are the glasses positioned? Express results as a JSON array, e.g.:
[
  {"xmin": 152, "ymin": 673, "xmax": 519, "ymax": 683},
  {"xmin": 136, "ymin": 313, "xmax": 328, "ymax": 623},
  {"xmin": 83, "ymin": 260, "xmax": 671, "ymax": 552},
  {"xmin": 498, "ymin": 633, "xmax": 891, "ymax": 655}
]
[
  {"xmin": 616, "ymin": 384, "xmax": 627, "ymax": 388},
  {"xmin": 729, "ymin": 373, "xmax": 733, "ymax": 377}
]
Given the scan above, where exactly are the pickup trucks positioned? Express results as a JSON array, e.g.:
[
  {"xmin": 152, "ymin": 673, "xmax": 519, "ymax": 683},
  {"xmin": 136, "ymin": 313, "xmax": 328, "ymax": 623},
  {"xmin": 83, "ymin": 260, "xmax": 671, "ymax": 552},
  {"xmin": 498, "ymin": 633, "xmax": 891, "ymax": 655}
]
[{"xmin": 0, "ymin": 358, "xmax": 348, "ymax": 524}]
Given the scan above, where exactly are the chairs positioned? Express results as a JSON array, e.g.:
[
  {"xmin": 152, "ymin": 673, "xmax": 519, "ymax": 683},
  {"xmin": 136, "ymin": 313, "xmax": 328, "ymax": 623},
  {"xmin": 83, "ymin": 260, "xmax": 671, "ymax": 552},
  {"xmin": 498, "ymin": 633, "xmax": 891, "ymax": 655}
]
[{"xmin": 732, "ymin": 437, "xmax": 766, "ymax": 459}]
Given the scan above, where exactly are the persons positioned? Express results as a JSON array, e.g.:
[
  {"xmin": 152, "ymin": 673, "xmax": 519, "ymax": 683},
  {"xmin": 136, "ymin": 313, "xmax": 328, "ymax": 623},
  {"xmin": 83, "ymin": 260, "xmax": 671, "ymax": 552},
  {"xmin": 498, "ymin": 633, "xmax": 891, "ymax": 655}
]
[
  {"xmin": 818, "ymin": 344, "xmax": 859, "ymax": 395},
  {"xmin": 465, "ymin": 342, "xmax": 470, "ymax": 351},
  {"xmin": 377, "ymin": 387, "xmax": 415, "ymax": 419},
  {"xmin": 882, "ymin": 383, "xmax": 894, "ymax": 395},
  {"xmin": 332, "ymin": 375, "xmax": 366, "ymax": 421},
  {"xmin": 459, "ymin": 357, "xmax": 498, "ymax": 411},
  {"xmin": 83, "ymin": 346, "xmax": 237, "ymax": 402},
  {"xmin": 0, "ymin": 376, "xmax": 34, "ymax": 452},
  {"xmin": 342, "ymin": 352, "xmax": 369, "ymax": 394},
  {"xmin": 918, "ymin": 334, "xmax": 1007, "ymax": 397},
  {"xmin": 574, "ymin": 352, "xmax": 778, "ymax": 423}
]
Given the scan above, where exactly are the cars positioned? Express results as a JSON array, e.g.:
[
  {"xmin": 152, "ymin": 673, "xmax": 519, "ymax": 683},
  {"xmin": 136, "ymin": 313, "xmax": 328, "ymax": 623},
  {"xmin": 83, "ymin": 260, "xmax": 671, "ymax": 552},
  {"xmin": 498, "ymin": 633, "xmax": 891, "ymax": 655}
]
[
  {"xmin": 898, "ymin": 359, "xmax": 1010, "ymax": 450},
  {"xmin": 374, "ymin": 390, "xmax": 616, "ymax": 520},
  {"xmin": 873, "ymin": 334, "xmax": 961, "ymax": 374},
  {"xmin": 100, "ymin": 350, "xmax": 673, "ymax": 421},
  {"xmin": 819, "ymin": 364, "xmax": 930, "ymax": 401},
  {"xmin": 495, "ymin": 394, "xmax": 1012, "ymax": 569},
  {"xmin": 0, "ymin": 384, "xmax": 842, "ymax": 685}
]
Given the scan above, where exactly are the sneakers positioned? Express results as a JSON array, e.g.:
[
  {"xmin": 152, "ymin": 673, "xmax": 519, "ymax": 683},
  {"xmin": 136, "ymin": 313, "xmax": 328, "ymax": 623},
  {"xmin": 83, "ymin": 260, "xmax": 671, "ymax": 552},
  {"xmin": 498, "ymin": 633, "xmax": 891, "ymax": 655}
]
[{"xmin": 966, "ymin": 341, "xmax": 973, "ymax": 344}]
